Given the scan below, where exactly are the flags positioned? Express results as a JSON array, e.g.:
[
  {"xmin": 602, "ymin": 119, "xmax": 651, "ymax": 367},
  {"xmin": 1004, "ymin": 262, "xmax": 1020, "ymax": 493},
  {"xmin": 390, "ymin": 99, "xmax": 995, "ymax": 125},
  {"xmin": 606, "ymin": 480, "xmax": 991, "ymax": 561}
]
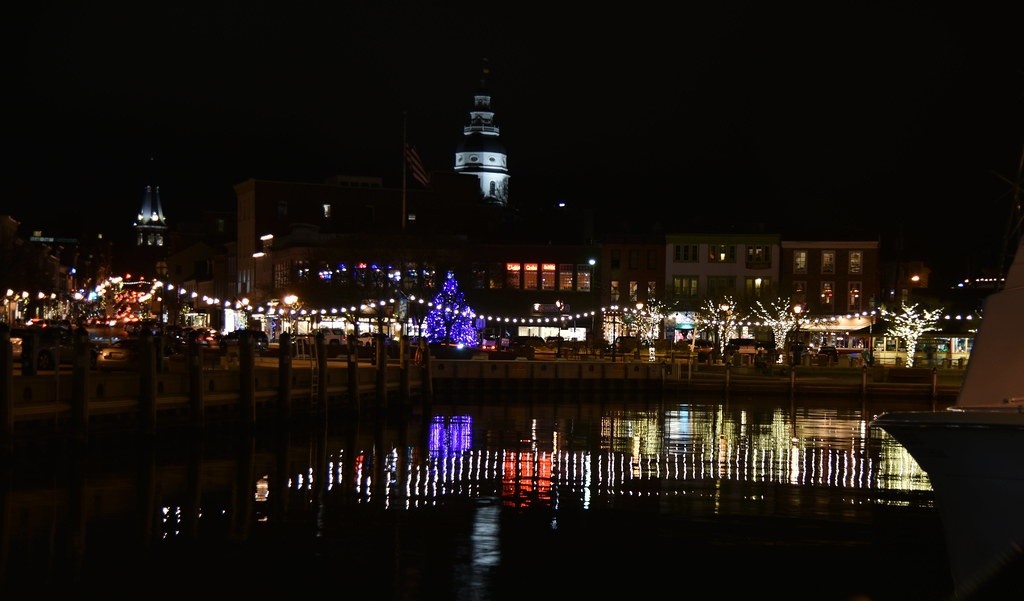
[{"xmin": 406, "ymin": 141, "xmax": 433, "ymax": 189}]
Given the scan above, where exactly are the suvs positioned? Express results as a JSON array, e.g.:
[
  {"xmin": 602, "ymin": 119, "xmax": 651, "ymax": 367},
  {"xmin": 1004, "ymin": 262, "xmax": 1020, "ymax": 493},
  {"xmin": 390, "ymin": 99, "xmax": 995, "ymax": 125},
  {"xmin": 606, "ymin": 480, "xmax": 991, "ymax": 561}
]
[
  {"xmin": 294, "ymin": 328, "xmax": 345, "ymax": 346},
  {"xmin": 219, "ymin": 330, "xmax": 268, "ymax": 349}
]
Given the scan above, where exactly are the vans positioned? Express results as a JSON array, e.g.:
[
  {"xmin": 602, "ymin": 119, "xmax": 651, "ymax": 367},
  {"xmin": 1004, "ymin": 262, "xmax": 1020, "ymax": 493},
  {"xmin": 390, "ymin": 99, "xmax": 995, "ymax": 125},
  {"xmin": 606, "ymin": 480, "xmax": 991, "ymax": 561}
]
[{"xmin": 510, "ymin": 336, "xmax": 545, "ymax": 347}]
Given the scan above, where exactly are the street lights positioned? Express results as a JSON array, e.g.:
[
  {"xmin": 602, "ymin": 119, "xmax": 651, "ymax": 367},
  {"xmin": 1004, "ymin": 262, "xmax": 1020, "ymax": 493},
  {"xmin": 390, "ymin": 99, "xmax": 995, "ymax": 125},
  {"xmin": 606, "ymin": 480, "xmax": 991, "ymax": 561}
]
[
  {"xmin": 793, "ymin": 305, "xmax": 802, "ymax": 365},
  {"xmin": 721, "ymin": 303, "xmax": 730, "ymax": 363},
  {"xmin": 556, "ymin": 299, "xmax": 565, "ymax": 358},
  {"xmin": 611, "ymin": 305, "xmax": 619, "ymax": 362},
  {"xmin": 635, "ymin": 302, "xmax": 643, "ymax": 359}
]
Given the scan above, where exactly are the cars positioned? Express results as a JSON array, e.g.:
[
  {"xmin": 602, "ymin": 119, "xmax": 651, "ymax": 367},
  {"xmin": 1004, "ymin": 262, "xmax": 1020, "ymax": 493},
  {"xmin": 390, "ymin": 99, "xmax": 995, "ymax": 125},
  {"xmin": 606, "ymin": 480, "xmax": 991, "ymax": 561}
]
[
  {"xmin": 125, "ymin": 318, "xmax": 221, "ymax": 345},
  {"xmin": 819, "ymin": 346, "xmax": 838, "ymax": 362},
  {"xmin": 483, "ymin": 337, "xmax": 497, "ymax": 350},
  {"xmin": 10, "ymin": 325, "xmax": 84, "ymax": 370},
  {"xmin": 97, "ymin": 339, "xmax": 144, "ymax": 369},
  {"xmin": 357, "ymin": 332, "xmax": 391, "ymax": 348},
  {"xmin": 790, "ymin": 342, "xmax": 813, "ymax": 351}
]
